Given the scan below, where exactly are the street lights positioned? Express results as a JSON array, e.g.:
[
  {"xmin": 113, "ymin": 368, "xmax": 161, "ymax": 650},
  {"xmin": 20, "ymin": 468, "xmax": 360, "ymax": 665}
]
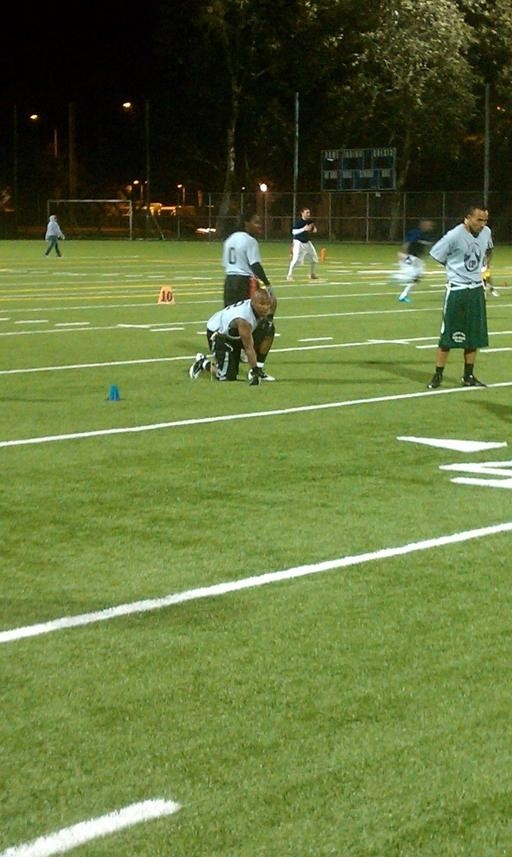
[
  {"xmin": 259, "ymin": 181, "xmax": 270, "ymax": 239},
  {"xmin": 122, "ymin": 98, "xmax": 151, "ymax": 202},
  {"xmin": 495, "ymin": 98, "xmax": 512, "ymax": 112},
  {"xmin": 29, "ymin": 110, "xmax": 61, "ymax": 201},
  {"xmin": 132, "ymin": 176, "xmax": 145, "ymax": 199},
  {"xmin": 177, "ymin": 183, "xmax": 186, "ymax": 204}
]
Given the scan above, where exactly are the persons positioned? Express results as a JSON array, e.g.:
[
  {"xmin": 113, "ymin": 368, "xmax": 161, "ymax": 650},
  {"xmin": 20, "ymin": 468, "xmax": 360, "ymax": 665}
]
[
  {"xmin": 286, "ymin": 207, "xmax": 322, "ymax": 283},
  {"xmin": 188, "ymin": 287, "xmax": 275, "ymax": 386},
  {"xmin": 43, "ymin": 215, "xmax": 65, "ymax": 259},
  {"xmin": 221, "ymin": 210, "xmax": 274, "ymax": 380},
  {"xmin": 395, "ymin": 219, "xmax": 434, "ymax": 304},
  {"xmin": 426, "ymin": 203, "xmax": 499, "ymax": 391}
]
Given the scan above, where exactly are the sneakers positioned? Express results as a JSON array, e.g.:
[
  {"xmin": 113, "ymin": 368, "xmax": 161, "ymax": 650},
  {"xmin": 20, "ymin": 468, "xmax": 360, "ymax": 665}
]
[
  {"xmin": 248, "ymin": 368, "xmax": 275, "ymax": 381},
  {"xmin": 189, "ymin": 352, "xmax": 205, "ymax": 379},
  {"xmin": 427, "ymin": 373, "xmax": 442, "ymax": 388},
  {"xmin": 461, "ymin": 374, "xmax": 485, "ymax": 387}
]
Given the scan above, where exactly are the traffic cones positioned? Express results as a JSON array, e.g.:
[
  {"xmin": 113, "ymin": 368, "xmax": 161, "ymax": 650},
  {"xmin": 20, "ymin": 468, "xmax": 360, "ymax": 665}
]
[{"xmin": 106, "ymin": 380, "xmax": 122, "ymax": 401}]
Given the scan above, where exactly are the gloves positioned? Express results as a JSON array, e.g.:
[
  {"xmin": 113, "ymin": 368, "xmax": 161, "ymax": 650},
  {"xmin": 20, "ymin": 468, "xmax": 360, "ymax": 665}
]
[{"xmin": 250, "ymin": 369, "xmax": 259, "ymax": 385}]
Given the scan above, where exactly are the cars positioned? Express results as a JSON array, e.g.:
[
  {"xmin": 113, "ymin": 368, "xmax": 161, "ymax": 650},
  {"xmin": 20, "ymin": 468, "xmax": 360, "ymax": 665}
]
[{"xmin": 119, "ymin": 202, "xmax": 177, "ymax": 216}]
